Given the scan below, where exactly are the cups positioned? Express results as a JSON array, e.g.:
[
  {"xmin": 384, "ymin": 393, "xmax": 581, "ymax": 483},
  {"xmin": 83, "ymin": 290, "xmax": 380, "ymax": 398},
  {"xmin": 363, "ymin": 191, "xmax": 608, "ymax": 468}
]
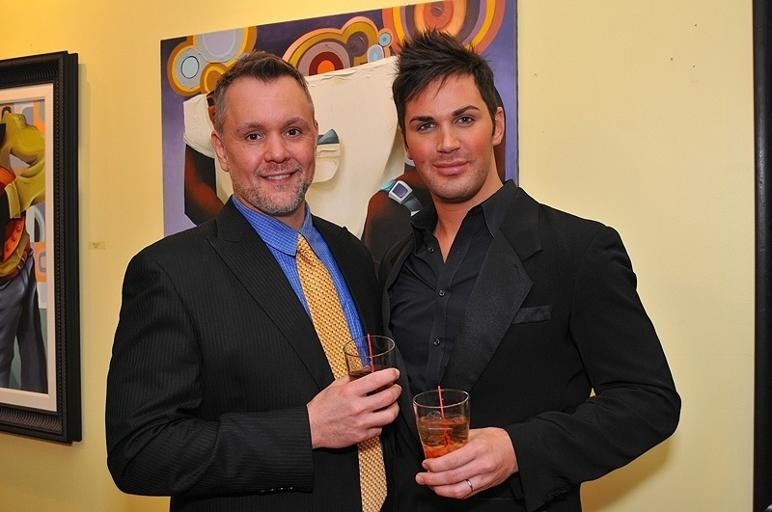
[
  {"xmin": 341, "ymin": 334, "xmax": 401, "ymax": 377},
  {"xmin": 413, "ymin": 388, "xmax": 473, "ymax": 467}
]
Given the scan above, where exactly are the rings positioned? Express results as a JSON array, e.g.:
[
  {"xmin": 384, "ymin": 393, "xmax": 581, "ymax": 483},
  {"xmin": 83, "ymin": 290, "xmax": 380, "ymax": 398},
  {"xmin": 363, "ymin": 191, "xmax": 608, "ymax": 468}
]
[{"xmin": 464, "ymin": 478, "xmax": 475, "ymax": 493}]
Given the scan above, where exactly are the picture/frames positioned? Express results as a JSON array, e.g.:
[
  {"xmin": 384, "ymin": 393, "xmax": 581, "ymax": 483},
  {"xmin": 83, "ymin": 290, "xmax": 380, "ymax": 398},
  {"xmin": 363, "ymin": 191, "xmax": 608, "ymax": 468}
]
[{"xmin": 0, "ymin": 52, "xmax": 83, "ymax": 444}]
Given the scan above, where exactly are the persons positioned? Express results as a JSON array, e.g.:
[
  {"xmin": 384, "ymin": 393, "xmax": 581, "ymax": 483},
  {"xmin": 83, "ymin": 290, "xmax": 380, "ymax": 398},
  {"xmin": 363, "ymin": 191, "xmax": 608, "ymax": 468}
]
[
  {"xmin": 105, "ymin": 51, "xmax": 403, "ymax": 512},
  {"xmin": 380, "ymin": 34, "xmax": 681, "ymax": 511}
]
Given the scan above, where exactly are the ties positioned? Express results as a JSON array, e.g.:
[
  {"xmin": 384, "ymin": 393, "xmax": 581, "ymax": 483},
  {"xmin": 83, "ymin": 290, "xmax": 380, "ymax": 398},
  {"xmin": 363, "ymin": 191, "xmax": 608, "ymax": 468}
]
[{"xmin": 297, "ymin": 236, "xmax": 388, "ymax": 512}]
